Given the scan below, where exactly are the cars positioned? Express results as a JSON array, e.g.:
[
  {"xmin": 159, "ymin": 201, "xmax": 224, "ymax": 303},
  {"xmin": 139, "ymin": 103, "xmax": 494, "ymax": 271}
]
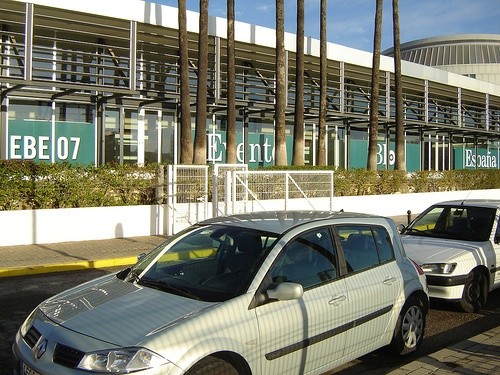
[
  {"xmin": 9, "ymin": 207, "xmax": 432, "ymax": 375},
  {"xmin": 396, "ymin": 191, "xmax": 500, "ymax": 313}
]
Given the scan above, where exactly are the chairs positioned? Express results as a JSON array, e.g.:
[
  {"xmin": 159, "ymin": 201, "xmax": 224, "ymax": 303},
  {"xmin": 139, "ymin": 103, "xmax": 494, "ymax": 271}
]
[
  {"xmin": 283, "ymin": 244, "xmax": 321, "ymax": 284},
  {"xmin": 343, "ymin": 234, "xmax": 378, "ymax": 272}
]
